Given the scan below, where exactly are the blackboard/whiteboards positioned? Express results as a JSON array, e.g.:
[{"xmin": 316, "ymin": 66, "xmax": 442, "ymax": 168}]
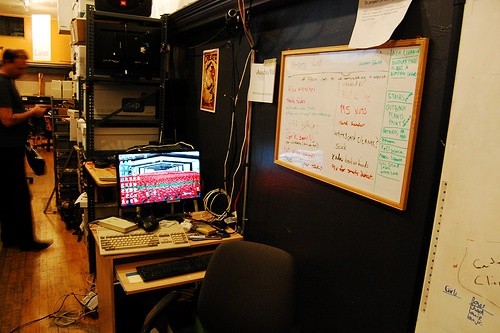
[{"xmin": 274, "ymin": 38, "xmax": 430, "ymax": 212}]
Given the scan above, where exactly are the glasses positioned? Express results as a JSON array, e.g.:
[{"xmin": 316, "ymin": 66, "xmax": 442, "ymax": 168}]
[{"xmin": 16, "ymin": 54, "xmax": 28, "ymax": 59}]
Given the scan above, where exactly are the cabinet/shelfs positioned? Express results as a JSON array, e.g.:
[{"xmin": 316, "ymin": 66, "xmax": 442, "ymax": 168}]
[
  {"xmin": 79, "ymin": 4, "xmax": 169, "ymax": 271},
  {"xmin": 50, "ymin": 97, "xmax": 81, "ymax": 212}
]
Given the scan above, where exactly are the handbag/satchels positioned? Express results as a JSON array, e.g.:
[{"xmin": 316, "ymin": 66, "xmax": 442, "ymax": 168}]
[{"xmin": 25, "ymin": 142, "xmax": 45, "ymax": 176}]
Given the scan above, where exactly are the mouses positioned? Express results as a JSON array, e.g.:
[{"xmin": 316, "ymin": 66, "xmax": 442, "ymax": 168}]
[
  {"xmin": 143, "ymin": 220, "xmax": 154, "ymax": 232},
  {"xmin": 212, "ymin": 220, "xmax": 227, "ymax": 229}
]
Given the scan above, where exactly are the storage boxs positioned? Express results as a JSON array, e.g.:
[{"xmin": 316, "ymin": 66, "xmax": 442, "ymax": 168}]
[{"xmin": 68, "ymin": 18, "xmax": 159, "ymax": 150}]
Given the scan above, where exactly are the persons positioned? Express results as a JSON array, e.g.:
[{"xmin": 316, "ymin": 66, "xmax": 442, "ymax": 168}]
[{"xmin": 0, "ymin": 49, "xmax": 54, "ymax": 251}]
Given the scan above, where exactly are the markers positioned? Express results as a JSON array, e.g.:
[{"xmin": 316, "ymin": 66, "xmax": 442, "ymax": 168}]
[{"xmin": 187, "ymin": 235, "xmax": 206, "ymax": 239}]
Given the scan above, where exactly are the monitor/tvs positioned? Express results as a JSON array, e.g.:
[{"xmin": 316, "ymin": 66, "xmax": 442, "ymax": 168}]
[{"xmin": 116, "ymin": 148, "xmax": 202, "ymax": 221}]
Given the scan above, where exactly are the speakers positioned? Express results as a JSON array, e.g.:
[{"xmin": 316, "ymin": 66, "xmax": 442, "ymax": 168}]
[{"xmin": 95, "ymin": 0, "xmax": 152, "ymax": 17}]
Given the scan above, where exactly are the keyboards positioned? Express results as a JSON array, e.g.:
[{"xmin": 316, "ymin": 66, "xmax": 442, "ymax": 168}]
[
  {"xmin": 135, "ymin": 254, "xmax": 214, "ymax": 281},
  {"xmin": 97, "ymin": 226, "xmax": 190, "ymax": 255}
]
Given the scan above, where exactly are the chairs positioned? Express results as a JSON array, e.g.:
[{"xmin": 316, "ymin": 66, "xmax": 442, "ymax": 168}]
[{"xmin": 146, "ymin": 240, "xmax": 293, "ymax": 333}]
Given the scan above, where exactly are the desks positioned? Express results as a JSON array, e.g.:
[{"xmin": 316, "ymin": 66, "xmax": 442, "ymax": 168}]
[{"xmin": 92, "ymin": 211, "xmax": 243, "ymax": 333}]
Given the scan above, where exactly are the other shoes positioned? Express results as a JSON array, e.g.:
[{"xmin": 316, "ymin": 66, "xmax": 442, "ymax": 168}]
[{"xmin": 21, "ymin": 239, "xmax": 54, "ymax": 251}]
